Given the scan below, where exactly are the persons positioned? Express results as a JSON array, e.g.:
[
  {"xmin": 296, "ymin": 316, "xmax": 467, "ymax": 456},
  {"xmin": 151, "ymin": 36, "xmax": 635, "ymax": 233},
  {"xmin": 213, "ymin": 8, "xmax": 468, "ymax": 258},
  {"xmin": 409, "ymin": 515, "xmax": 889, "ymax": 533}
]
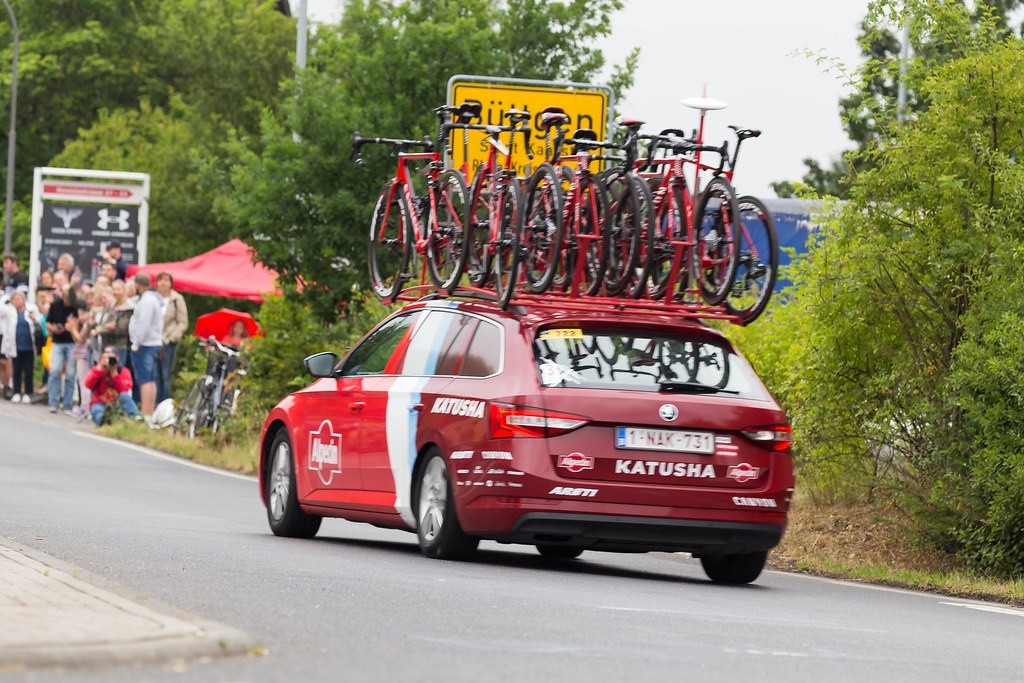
[
  {"xmin": 0, "ymin": 242, "xmax": 188, "ymax": 428},
  {"xmin": 217, "ymin": 319, "xmax": 252, "ymax": 403}
]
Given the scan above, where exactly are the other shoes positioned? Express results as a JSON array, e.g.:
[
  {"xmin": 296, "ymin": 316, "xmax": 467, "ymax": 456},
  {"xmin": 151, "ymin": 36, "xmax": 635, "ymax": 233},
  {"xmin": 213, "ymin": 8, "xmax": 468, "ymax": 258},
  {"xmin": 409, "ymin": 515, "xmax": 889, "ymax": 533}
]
[
  {"xmin": 144, "ymin": 415, "xmax": 160, "ymax": 428},
  {"xmin": 49, "ymin": 406, "xmax": 56, "ymax": 413},
  {"xmin": 11, "ymin": 393, "xmax": 21, "ymax": 402},
  {"xmin": 22, "ymin": 394, "xmax": 31, "ymax": 404},
  {"xmin": 62, "ymin": 410, "xmax": 71, "ymax": 415}
]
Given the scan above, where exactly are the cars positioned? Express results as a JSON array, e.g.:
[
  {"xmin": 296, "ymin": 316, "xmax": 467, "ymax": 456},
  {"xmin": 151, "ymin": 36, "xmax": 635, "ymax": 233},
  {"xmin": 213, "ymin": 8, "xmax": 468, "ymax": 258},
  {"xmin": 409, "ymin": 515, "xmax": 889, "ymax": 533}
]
[{"xmin": 259, "ymin": 284, "xmax": 795, "ymax": 585}]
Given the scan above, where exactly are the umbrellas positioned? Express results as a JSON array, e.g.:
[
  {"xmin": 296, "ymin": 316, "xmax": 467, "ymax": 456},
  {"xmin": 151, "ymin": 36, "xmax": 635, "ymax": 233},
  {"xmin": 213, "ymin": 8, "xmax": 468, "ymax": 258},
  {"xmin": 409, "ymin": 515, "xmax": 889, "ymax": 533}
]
[{"xmin": 193, "ymin": 308, "xmax": 264, "ymax": 344}]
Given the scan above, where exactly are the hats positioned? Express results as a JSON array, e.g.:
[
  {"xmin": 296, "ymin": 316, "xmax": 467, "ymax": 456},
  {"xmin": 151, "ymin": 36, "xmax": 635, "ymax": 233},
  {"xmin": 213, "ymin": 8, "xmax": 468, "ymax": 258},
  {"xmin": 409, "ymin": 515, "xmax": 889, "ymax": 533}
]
[{"xmin": 107, "ymin": 242, "xmax": 121, "ymax": 251}]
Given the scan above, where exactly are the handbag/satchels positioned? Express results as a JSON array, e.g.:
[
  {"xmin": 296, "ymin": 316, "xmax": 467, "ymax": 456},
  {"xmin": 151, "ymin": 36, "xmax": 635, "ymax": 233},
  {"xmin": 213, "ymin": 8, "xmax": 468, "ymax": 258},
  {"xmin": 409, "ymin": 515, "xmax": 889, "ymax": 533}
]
[{"xmin": 42, "ymin": 337, "xmax": 65, "ymax": 374}]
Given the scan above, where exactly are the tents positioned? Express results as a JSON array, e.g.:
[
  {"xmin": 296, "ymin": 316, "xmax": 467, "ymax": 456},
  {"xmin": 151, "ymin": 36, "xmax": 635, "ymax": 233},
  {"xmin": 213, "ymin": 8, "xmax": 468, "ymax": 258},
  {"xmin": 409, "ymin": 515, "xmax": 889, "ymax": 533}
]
[{"xmin": 125, "ymin": 238, "xmax": 349, "ymax": 308}]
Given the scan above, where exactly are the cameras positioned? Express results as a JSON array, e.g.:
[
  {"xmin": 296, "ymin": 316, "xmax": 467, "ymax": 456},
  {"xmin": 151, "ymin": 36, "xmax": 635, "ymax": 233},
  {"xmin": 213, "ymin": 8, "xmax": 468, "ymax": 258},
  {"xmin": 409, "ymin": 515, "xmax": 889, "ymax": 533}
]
[{"xmin": 107, "ymin": 357, "xmax": 117, "ymax": 365}]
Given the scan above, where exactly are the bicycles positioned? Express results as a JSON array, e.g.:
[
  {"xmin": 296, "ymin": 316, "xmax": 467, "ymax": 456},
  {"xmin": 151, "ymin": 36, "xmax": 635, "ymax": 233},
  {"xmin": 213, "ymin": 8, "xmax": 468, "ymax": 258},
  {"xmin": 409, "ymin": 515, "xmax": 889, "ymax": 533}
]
[
  {"xmin": 170, "ymin": 335, "xmax": 247, "ymax": 440},
  {"xmin": 349, "ymin": 104, "xmax": 778, "ymax": 326}
]
